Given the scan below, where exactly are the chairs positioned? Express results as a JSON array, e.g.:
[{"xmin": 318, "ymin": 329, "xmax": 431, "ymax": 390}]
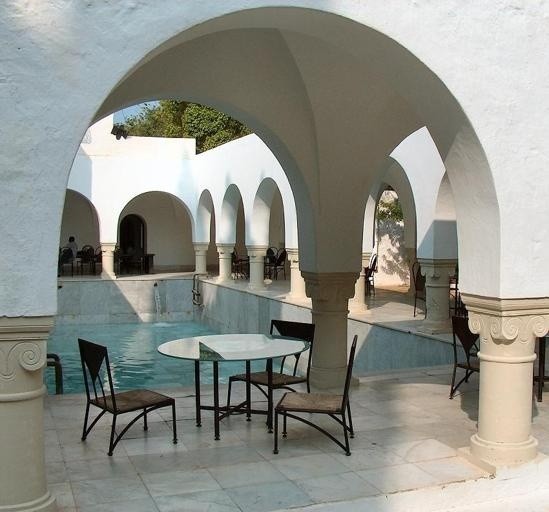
[
  {"xmin": 448, "ymin": 315, "xmax": 480, "ymax": 399},
  {"xmin": 57, "ymin": 243, "xmax": 150, "ymax": 278},
  {"xmin": 264, "ymin": 247, "xmax": 287, "ymax": 280},
  {"xmin": 363, "ymin": 254, "xmax": 378, "ymax": 298},
  {"xmin": 77, "ymin": 317, "xmax": 359, "ymax": 456},
  {"xmin": 411, "ymin": 261, "xmax": 427, "ymax": 318},
  {"xmin": 232, "ymin": 253, "xmax": 249, "ymax": 281}
]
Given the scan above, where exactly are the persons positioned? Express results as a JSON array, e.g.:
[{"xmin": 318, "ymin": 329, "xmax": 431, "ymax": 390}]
[{"xmin": 66, "ymin": 235, "xmax": 79, "ymax": 260}]
